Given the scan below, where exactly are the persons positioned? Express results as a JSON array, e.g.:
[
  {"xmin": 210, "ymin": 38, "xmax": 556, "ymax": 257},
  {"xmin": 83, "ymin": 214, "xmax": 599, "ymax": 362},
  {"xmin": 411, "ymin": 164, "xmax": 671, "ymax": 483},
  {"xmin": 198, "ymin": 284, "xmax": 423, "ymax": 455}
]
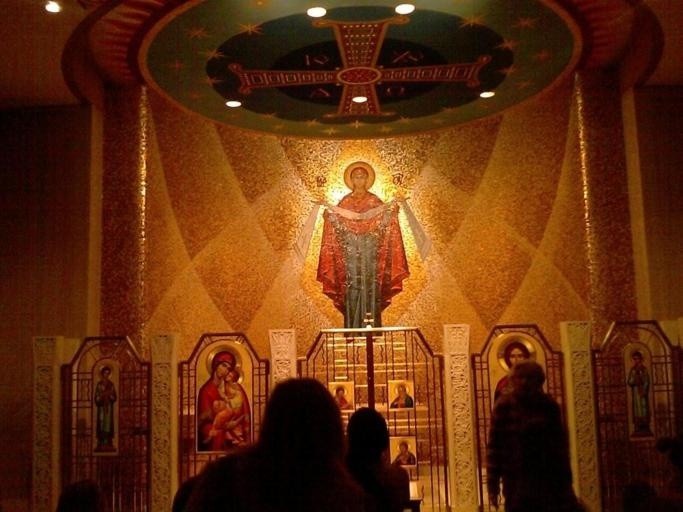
[
  {"xmin": 196, "ymin": 349, "xmax": 235, "ymax": 451},
  {"xmin": 391, "ymin": 442, "xmax": 415, "ymax": 464},
  {"xmin": 626, "ymin": 350, "xmax": 652, "ymax": 436},
  {"xmin": 205, "ymin": 369, "xmax": 251, "ymax": 441},
  {"xmin": 171, "ymin": 474, "xmax": 195, "ymax": 511},
  {"xmin": 485, "ymin": 359, "xmax": 584, "ymax": 511},
  {"xmin": 389, "ymin": 384, "xmax": 413, "ymax": 407},
  {"xmin": 344, "ymin": 406, "xmax": 409, "ymax": 510},
  {"xmin": 331, "ymin": 387, "xmax": 352, "ymax": 409},
  {"xmin": 622, "ymin": 481, "xmax": 659, "ymax": 511},
  {"xmin": 656, "ymin": 436, "xmax": 683, "ymax": 491},
  {"xmin": 495, "ymin": 342, "xmax": 529, "ymax": 403},
  {"xmin": 310, "ymin": 161, "xmax": 412, "ymax": 337},
  {"xmin": 55, "ymin": 479, "xmax": 109, "ymax": 511},
  {"xmin": 182, "ymin": 375, "xmax": 365, "ymax": 510},
  {"xmin": 92, "ymin": 364, "xmax": 117, "ymax": 452}
]
